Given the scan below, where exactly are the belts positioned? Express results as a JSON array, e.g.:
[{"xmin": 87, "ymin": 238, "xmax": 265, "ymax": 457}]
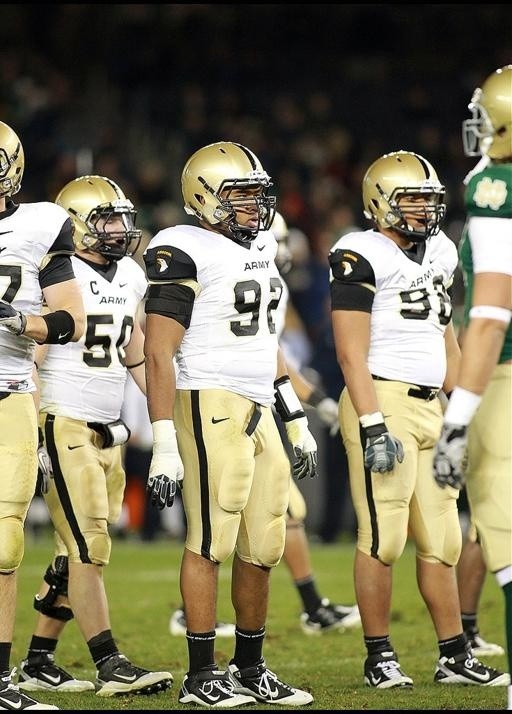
[
  {"xmin": 0, "ymin": 391, "xmax": 12, "ymax": 401},
  {"xmin": 245, "ymin": 402, "xmax": 262, "ymax": 436},
  {"xmin": 370, "ymin": 375, "xmax": 439, "ymax": 400}
]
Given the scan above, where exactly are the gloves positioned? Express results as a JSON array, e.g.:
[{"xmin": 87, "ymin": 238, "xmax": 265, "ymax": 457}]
[
  {"xmin": 33, "ymin": 444, "xmax": 55, "ymax": 496},
  {"xmin": 146, "ymin": 451, "xmax": 185, "ymax": 511},
  {"xmin": 361, "ymin": 422, "xmax": 405, "ymax": 473},
  {"xmin": 287, "ymin": 427, "xmax": 318, "ymax": 480},
  {"xmin": 316, "ymin": 395, "xmax": 342, "ymax": 436},
  {"xmin": 0, "ymin": 299, "xmax": 27, "ymax": 336},
  {"xmin": 433, "ymin": 421, "xmax": 471, "ymax": 491}
]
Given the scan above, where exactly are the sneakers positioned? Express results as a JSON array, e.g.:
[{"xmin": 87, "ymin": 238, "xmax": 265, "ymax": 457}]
[
  {"xmin": 94, "ymin": 654, "xmax": 175, "ymax": 697},
  {"xmin": 463, "ymin": 630, "xmax": 505, "ymax": 657},
  {"xmin": 0, "ymin": 670, "xmax": 59, "ymax": 710},
  {"xmin": 167, "ymin": 609, "xmax": 237, "ymax": 639},
  {"xmin": 433, "ymin": 640, "xmax": 511, "ymax": 686},
  {"xmin": 360, "ymin": 646, "xmax": 414, "ymax": 689},
  {"xmin": 226, "ymin": 659, "xmax": 315, "ymax": 707},
  {"xmin": 16, "ymin": 652, "xmax": 96, "ymax": 694},
  {"xmin": 177, "ymin": 663, "xmax": 257, "ymax": 709},
  {"xmin": 300, "ymin": 601, "xmax": 364, "ymax": 634}
]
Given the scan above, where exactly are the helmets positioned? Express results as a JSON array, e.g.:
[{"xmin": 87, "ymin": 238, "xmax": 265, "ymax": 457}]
[
  {"xmin": 1, "ymin": 121, "xmax": 25, "ymax": 199},
  {"xmin": 180, "ymin": 140, "xmax": 267, "ymax": 225},
  {"xmin": 56, "ymin": 174, "xmax": 136, "ymax": 251},
  {"xmin": 475, "ymin": 63, "xmax": 512, "ymax": 159},
  {"xmin": 362, "ymin": 150, "xmax": 446, "ymax": 230},
  {"xmin": 260, "ymin": 205, "xmax": 289, "ymax": 243}
]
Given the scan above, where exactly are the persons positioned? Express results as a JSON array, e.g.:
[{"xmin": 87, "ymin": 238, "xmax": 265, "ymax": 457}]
[{"xmin": 1, "ymin": 2, "xmax": 512, "ymax": 711}]
